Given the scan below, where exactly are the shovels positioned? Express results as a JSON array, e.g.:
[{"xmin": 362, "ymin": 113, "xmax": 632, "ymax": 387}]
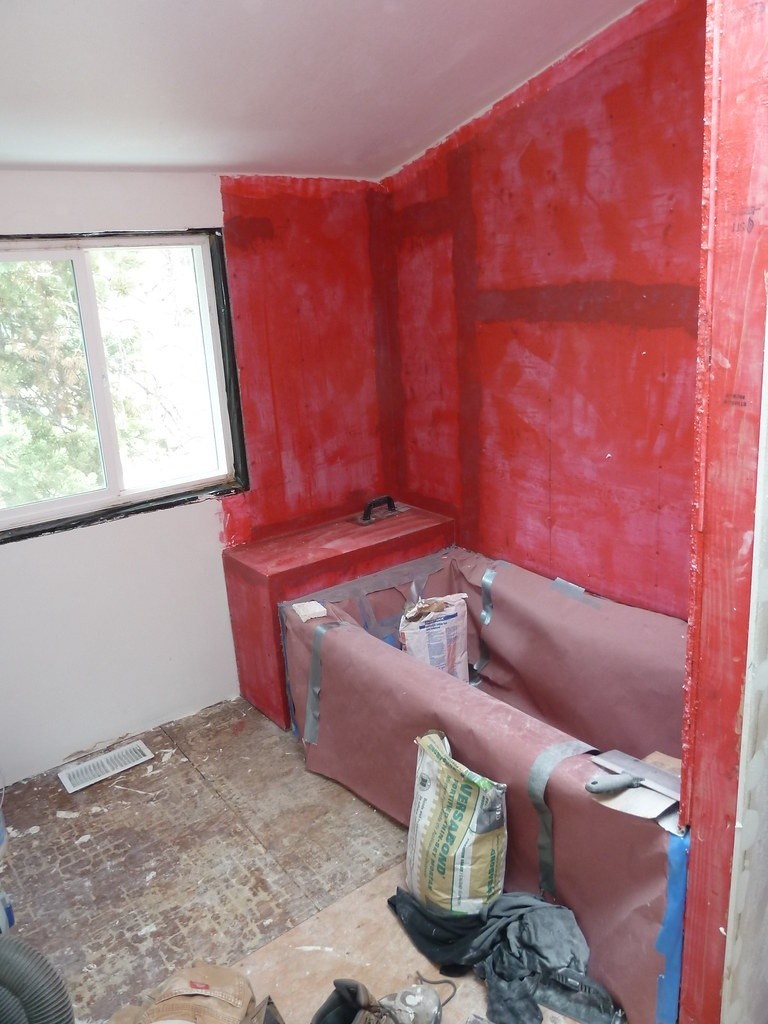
[{"xmin": 585, "ymin": 749, "xmax": 681, "ymax": 802}]
